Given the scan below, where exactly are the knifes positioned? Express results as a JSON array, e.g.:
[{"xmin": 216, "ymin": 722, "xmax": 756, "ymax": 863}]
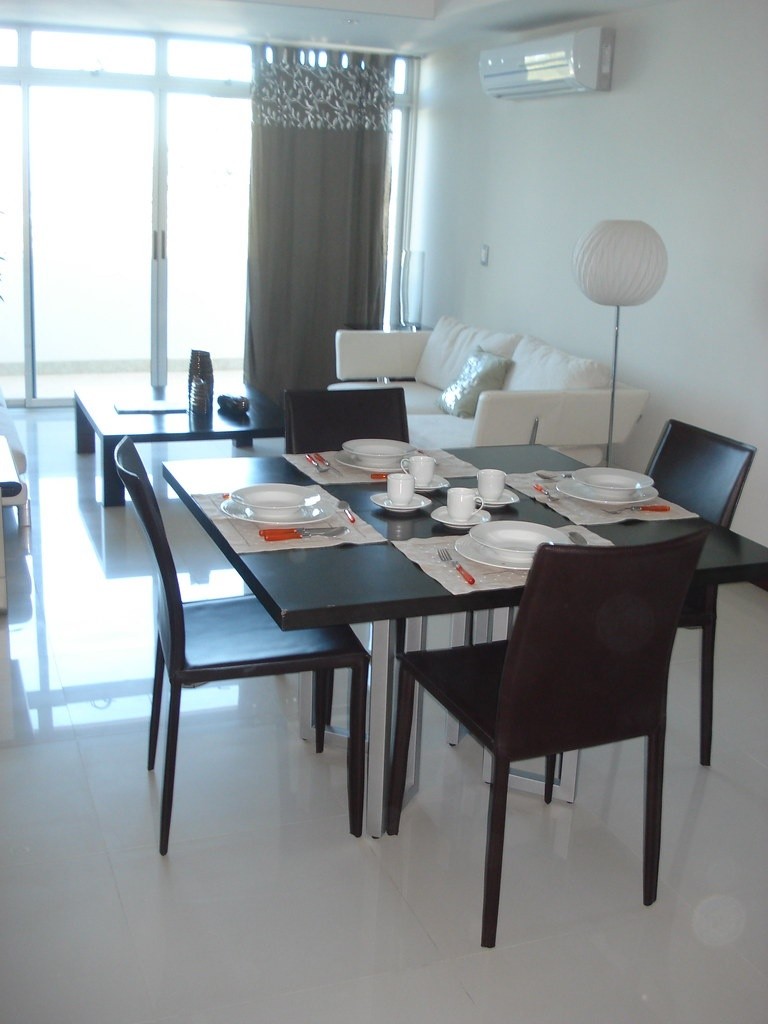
[
  {"xmin": 314, "ymin": 453, "xmax": 342, "ymax": 476},
  {"xmin": 259, "ymin": 527, "xmax": 336, "ymax": 536}
]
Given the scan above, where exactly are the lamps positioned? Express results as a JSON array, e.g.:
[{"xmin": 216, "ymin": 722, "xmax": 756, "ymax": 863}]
[{"xmin": 573, "ymin": 219, "xmax": 668, "ymax": 468}]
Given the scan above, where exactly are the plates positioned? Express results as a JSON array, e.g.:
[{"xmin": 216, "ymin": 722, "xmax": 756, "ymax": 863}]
[
  {"xmin": 370, "ymin": 493, "xmax": 432, "ymax": 513},
  {"xmin": 470, "ymin": 488, "xmax": 519, "ymax": 507},
  {"xmin": 414, "ymin": 475, "xmax": 450, "ymax": 492},
  {"xmin": 454, "ymin": 534, "xmax": 533, "ymax": 570},
  {"xmin": 221, "ymin": 499, "xmax": 335, "ymax": 526},
  {"xmin": 430, "ymin": 506, "xmax": 491, "ymax": 528},
  {"xmin": 556, "ymin": 478, "xmax": 658, "ymax": 509},
  {"xmin": 335, "ymin": 450, "xmax": 424, "ymax": 473}
]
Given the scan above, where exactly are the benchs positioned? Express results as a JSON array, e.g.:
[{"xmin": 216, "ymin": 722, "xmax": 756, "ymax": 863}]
[
  {"xmin": 327, "ymin": 329, "xmax": 650, "ymax": 465},
  {"xmin": 0, "ymin": 390, "xmax": 30, "ymax": 527}
]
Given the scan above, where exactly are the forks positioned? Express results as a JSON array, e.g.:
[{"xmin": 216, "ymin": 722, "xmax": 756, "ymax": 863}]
[
  {"xmin": 438, "ymin": 549, "xmax": 475, "ymax": 584},
  {"xmin": 600, "ymin": 505, "xmax": 670, "ymax": 514}
]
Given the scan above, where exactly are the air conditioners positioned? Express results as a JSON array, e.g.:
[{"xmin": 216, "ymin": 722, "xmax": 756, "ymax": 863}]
[{"xmin": 478, "ymin": 26, "xmax": 615, "ymax": 98}]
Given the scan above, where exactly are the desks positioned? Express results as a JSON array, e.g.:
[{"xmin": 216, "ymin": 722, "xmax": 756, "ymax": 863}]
[{"xmin": 163, "ymin": 444, "xmax": 767, "ymax": 840}]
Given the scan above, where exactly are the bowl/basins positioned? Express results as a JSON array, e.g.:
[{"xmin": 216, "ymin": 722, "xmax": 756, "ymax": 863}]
[
  {"xmin": 230, "ymin": 483, "xmax": 321, "ymax": 518},
  {"xmin": 342, "ymin": 439, "xmax": 417, "ymax": 465},
  {"xmin": 468, "ymin": 520, "xmax": 570, "ymax": 564},
  {"xmin": 572, "ymin": 467, "xmax": 655, "ymax": 501}
]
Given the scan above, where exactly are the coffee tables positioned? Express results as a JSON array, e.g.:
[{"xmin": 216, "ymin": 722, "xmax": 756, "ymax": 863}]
[{"xmin": 73, "ymin": 385, "xmax": 285, "ymax": 507}]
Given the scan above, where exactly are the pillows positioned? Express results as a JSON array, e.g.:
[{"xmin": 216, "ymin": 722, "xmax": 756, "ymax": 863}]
[{"xmin": 438, "ymin": 344, "xmax": 515, "ymax": 420}]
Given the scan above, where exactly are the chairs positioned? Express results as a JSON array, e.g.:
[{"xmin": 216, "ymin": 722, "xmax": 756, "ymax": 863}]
[
  {"xmin": 283, "ymin": 387, "xmax": 410, "ymax": 455},
  {"xmin": 386, "ymin": 527, "xmax": 710, "ymax": 949},
  {"xmin": 646, "ymin": 419, "xmax": 757, "ymax": 766},
  {"xmin": 114, "ymin": 435, "xmax": 373, "ymax": 855}
]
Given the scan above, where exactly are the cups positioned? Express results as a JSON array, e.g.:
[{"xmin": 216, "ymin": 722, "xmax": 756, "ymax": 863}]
[
  {"xmin": 400, "ymin": 456, "xmax": 435, "ymax": 486},
  {"xmin": 477, "ymin": 468, "xmax": 507, "ymax": 502},
  {"xmin": 447, "ymin": 487, "xmax": 484, "ymax": 522},
  {"xmin": 387, "ymin": 519, "xmax": 414, "ymax": 542},
  {"xmin": 387, "ymin": 474, "xmax": 414, "ymax": 506}
]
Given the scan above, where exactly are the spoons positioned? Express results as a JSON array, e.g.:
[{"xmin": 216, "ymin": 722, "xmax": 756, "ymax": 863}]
[
  {"xmin": 568, "ymin": 532, "xmax": 586, "ymax": 546},
  {"xmin": 534, "ymin": 484, "xmax": 559, "ymax": 501},
  {"xmin": 536, "ymin": 472, "xmax": 572, "ymax": 479},
  {"xmin": 264, "ymin": 526, "xmax": 348, "ymax": 542},
  {"xmin": 305, "ymin": 453, "xmax": 330, "ymax": 473},
  {"xmin": 337, "ymin": 501, "xmax": 355, "ymax": 524}
]
